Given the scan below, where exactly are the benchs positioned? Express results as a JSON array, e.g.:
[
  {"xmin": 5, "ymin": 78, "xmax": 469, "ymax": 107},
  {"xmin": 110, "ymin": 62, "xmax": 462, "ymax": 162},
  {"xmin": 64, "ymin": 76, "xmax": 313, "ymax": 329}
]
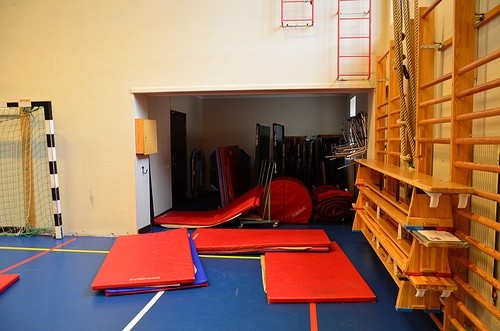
[{"xmin": 351, "ymin": 159, "xmax": 475, "ymax": 314}]
